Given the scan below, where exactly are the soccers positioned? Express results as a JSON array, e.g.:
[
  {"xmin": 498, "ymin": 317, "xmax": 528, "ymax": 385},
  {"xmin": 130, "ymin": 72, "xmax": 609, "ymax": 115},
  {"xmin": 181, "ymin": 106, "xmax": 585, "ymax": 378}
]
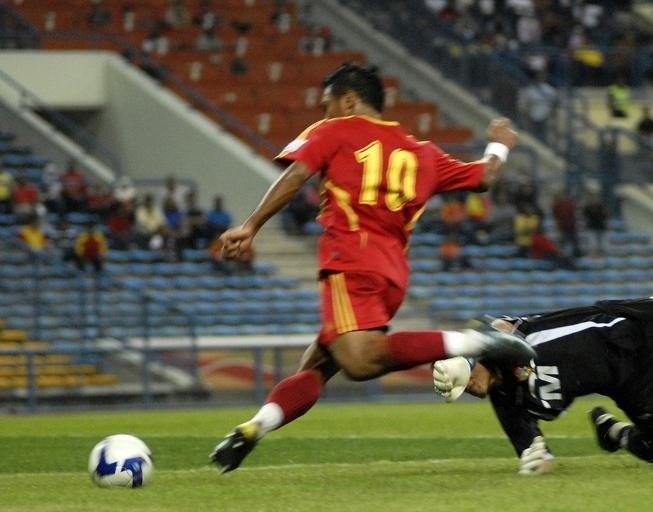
[{"xmin": 88, "ymin": 431, "xmax": 154, "ymax": 491}]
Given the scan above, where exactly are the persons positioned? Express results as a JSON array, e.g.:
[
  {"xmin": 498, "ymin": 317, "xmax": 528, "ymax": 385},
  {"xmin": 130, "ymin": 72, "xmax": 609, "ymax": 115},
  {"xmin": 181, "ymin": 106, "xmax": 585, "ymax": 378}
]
[
  {"xmin": 0, "ymin": 159, "xmax": 230, "ymax": 274},
  {"xmin": 426, "ymin": 0, "xmax": 653, "ymax": 136},
  {"xmin": 428, "ymin": 297, "xmax": 653, "ymax": 476},
  {"xmin": 442, "ymin": 177, "xmax": 608, "ymax": 272},
  {"xmin": 142, "ymin": 1, "xmax": 227, "ymax": 56},
  {"xmin": 208, "ymin": 59, "xmax": 538, "ymax": 478}
]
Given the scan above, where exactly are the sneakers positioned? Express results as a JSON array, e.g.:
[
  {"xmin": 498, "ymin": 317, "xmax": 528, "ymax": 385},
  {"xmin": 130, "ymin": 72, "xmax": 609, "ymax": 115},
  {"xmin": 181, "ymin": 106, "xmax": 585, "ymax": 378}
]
[
  {"xmin": 468, "ymin": 329, "xmax": 536, "ymax": 360},
  {"xmin": 209, "ymin": 422, "xmax": 260, "ymax": 473},
  {"xmin": 592, "ymin": 406, "xmax": 620, "ymax": 451}
]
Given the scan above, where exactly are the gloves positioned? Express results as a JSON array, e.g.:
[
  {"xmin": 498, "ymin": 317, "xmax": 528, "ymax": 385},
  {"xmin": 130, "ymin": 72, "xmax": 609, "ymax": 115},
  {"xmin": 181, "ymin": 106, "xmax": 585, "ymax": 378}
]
[
  {"xmin": 519, "ymin": 443, "xmax": 547, "ymax": 474},
  {"xmin": 433, "ymin": 356, "xmax": 471, "ymax": 400}
]
